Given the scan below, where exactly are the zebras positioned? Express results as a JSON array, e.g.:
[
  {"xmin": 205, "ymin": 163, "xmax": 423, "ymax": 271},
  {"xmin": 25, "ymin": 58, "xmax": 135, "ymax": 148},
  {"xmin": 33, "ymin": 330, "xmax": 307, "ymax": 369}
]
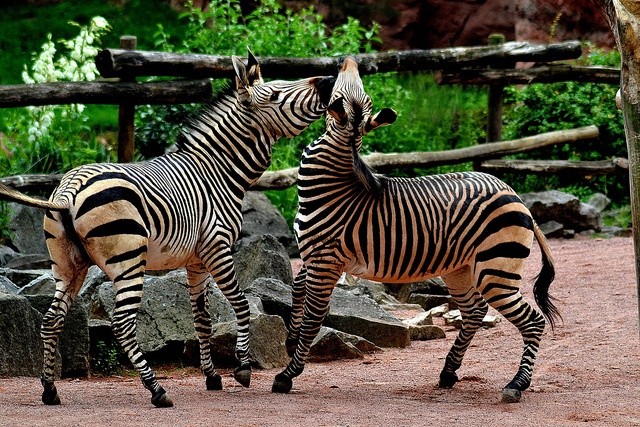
[
  {"xmin": 272, "ymin": 54, "xmax": 563, "ymax": 401},
  {"xmin": 0, "ymin": 44, "xmax": 335, "ymax": 408}
]
